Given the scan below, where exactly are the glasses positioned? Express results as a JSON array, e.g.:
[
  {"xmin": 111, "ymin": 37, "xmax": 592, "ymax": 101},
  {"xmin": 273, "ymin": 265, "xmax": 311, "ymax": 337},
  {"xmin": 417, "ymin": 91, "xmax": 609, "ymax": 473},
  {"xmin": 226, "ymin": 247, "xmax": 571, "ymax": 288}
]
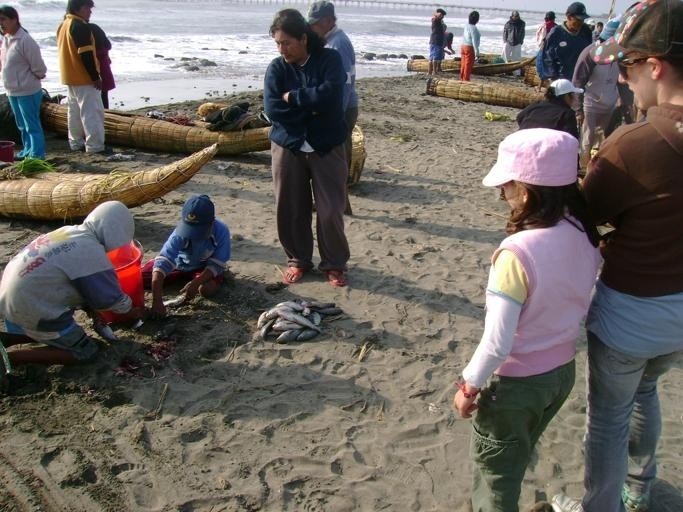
[
  {"xmin": 545, "ymin": 18, "xmax": 553, "ymax": 21},
  {"xmin": 617, "ymin": 58, "xmax": 647, "ymax": 80}
]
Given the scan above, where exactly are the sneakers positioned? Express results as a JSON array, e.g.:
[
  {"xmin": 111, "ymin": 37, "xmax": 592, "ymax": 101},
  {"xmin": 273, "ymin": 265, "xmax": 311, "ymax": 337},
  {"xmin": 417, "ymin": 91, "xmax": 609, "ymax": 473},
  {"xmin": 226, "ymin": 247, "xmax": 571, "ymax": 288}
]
[
  {"xmin": 97, "ymin": 146, "xmax": 113, "ymax": 155},
  {"xmin": 551, "ymin": 494, "xmax": 585, "ymax": 512},
  {"xmin": 621, "ymin": 482, "xmax": 653, "ymax": 511}
]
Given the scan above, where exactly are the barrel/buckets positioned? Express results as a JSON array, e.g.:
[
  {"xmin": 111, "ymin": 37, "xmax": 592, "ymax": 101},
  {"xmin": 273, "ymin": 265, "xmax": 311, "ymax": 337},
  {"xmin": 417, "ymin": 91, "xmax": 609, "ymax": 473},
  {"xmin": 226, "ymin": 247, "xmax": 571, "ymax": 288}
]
[
  {"xmin": 94, "ymin": 237, "xmax": 145, "ymax": 323},
  {"xmin": 0, "ymin": 140, "xmax": 16, "ymax": 162}
]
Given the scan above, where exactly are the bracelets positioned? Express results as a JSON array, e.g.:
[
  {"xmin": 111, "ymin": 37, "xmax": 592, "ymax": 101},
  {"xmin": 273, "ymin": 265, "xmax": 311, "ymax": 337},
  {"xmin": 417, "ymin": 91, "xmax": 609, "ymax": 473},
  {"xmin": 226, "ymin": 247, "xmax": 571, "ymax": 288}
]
[{"xmin": 461, "ymin": 384, "xmax": 479, "ymax": 397}]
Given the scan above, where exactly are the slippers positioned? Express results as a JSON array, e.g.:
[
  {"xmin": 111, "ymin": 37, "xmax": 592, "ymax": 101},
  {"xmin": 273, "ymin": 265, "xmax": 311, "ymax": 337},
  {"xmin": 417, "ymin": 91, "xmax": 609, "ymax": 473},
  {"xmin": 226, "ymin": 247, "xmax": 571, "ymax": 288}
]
[
  {"xmin": 286, "ymin": 266, "xmax": 304, "ymax": 283},
  {"xmin": 326, "ymin": 270, "xmax": 347, "ymax": 286}
]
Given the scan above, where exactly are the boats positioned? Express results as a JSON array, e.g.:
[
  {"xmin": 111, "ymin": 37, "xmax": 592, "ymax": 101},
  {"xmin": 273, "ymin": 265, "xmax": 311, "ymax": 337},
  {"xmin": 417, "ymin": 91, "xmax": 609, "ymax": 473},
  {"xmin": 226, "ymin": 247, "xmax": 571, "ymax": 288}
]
[
  {"xmin": 406, "ymin": 55, "xmax": 538, "ymax": 72},
  {"xmin": 1, "ymin": 139, "xmax": 222, "ymax": 221},
  {"xmin": 521, "ymin": 62, "xmax": 552, "ymax": 90},
  {"xmin": 23, "ymin": 97, "xmax": 270, "ymax": 158},
  {"xmin": 426, "ymin": 77, "xmax": 546, "ymax": 110}
]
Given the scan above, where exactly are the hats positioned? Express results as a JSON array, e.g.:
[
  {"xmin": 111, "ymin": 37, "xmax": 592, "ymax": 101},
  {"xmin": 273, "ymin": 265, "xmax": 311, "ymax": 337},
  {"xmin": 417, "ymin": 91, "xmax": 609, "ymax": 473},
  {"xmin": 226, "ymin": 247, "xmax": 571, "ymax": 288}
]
[
  {"xmin": 482, "ymin": 128, "xmax": 578, "ymax": 186},
  {"xmin": 307, "ymin": 1, "xmax": 334, "ymax": 24},
  {"xmin": 590, "ymin": 0, "xmax": 682, "ymax": 65},
  {"xmin": 550, "ymin": 78, "xmax": 584, "ymax": 96},
  {"xmin": 567, "ymin": 2, "xmax": 590, "ymax": 19},
  {"xmin": 512, "ymin": 10, "xmax": 519, "ymax": 16},
  {"xmin": 175, "ymin": 195, "xmax": 214, "ymax": 241},
  {"xmin": 437, "ymin": 9, "xmax": 446, "ymax": 15},
  {"xmin": 546, "ymin": 12, "xmax": 556, "ymax": 18}
]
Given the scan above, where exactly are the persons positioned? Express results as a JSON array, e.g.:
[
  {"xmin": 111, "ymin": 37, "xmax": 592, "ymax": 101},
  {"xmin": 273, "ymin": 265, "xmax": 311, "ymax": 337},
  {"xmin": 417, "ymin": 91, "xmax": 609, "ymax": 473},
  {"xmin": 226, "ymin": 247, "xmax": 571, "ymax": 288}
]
[
  {"xmin": 459, "ymin": 12, "xmax": 481, "ymax": 82},
  {"xmin": 303, "ymin": 3, "xmax": 359, "ymax": 215},
  {"xmin": 451, "ymin": 127, "xmax": 602, "ymax": 512},
  {"xmin": 263, "ymin": 8, "xmax": 350, "ymax": 287},
  {"xmin": 426, "ymin": 9, "xmax": 457, "ymax": 76},
  {"xmin": 500, "ymin": 11, "xmax": 526, "ymax": 76},
  {"xmin": 1, "ymin": 4, "xmax": 47, "ymax": 161},
  {"xmin": 142, "ymin": 194, "xmax": 231, "ymax": 320},
  {"xmin": 0, "ymin": 200, "xmax": 149, "ymax": 387},
  {"xmin": 552, "ymin": 0, "xmax": 683, "ymax": 512},
  {"xmin": 57, "ymin": 0, "xmax": 113, "ymax": 155},
  {"xmin": 513, "ymin": 1, "xmax": 651, "ymax": 188}
]
